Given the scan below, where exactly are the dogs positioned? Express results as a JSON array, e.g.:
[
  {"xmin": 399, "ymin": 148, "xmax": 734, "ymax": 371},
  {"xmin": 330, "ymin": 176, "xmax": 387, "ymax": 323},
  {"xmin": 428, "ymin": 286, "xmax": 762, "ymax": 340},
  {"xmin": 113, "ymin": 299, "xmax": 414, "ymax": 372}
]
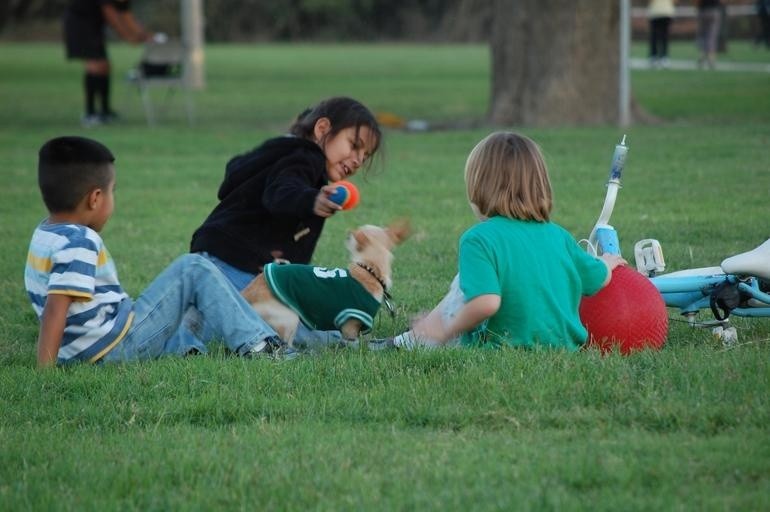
[{"xmin": 235, "ymin": 220, "xmax": 411, "ymax": 349}]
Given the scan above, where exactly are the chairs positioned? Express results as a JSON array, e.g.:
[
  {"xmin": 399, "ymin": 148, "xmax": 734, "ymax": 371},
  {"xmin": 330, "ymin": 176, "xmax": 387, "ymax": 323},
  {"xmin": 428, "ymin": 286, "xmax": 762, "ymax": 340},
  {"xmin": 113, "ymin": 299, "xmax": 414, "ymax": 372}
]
[{"xmin": 120, "ymin": 31, "xmax": 195, "ymax": 127}]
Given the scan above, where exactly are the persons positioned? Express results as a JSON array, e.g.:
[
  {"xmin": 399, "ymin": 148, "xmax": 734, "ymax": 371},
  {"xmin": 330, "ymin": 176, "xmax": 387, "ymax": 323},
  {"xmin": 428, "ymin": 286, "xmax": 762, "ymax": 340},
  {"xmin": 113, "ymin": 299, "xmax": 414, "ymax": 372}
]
[
  {"xmin": 61, "ymin": 0, "xmax": 153, "ymax": 124},
  {"xmin": 695, "ymin": 0, "xmax": 724, "ymax": 71},
  {"xmin": 384, "ymin": 128, "xmax": 627, "ymax": 352},
  {"xmin": 190, "ymin": 96, "xmax": 395, "ymax": 349},
  {"xmin": 645, "ymin": 0, "xmax": 678, "ymax": 70},
  {"xmin": 24, "ymin": 134, "xmax": 299, "ymax": 367}
]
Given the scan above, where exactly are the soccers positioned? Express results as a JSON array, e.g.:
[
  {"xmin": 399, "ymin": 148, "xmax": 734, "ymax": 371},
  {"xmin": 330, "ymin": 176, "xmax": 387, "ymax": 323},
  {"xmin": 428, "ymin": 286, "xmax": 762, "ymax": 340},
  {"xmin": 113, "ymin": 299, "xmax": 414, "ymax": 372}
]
[
  {"xmin": 330, "ymin": 181, "xmax": 359, "ymax": 209},
  {"xmin": 579, "ymin": 266, "xmax": 668, "ymax": 354}
]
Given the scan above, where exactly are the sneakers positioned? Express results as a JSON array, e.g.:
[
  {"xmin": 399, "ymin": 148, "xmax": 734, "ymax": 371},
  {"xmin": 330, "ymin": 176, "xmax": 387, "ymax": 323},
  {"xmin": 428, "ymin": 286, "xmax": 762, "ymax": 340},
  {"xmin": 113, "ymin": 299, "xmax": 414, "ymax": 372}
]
[{"xmin": 242, "ymin": 335, "xmax": 309, "ymax": 362}]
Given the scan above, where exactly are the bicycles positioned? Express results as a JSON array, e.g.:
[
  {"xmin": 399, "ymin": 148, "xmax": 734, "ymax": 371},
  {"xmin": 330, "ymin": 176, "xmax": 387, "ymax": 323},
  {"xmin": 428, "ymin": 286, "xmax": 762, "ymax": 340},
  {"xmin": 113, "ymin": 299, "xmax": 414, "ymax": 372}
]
[{"xmin": 577, "ymin": 134, "xmax": 770, "ymax": 344}]
